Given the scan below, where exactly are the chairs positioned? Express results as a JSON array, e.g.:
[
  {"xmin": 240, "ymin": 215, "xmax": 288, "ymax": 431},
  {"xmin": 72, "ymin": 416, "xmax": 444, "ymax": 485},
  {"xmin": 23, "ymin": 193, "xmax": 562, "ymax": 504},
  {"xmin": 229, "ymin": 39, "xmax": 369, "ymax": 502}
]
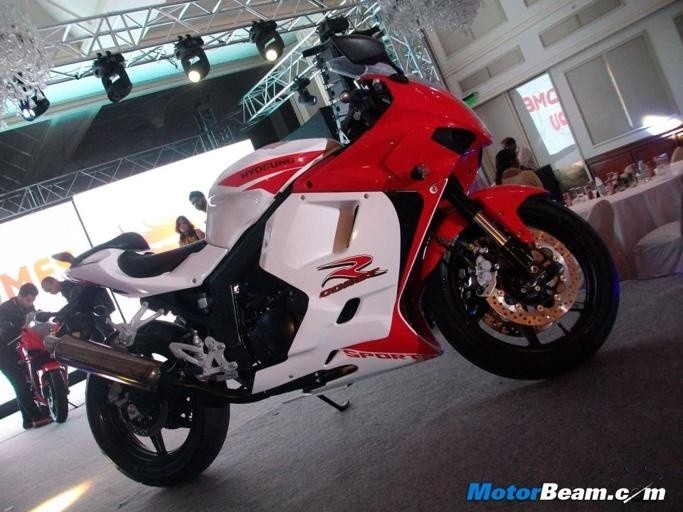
[{"xmin": 585, "ymin": 197, "xmax": 683, "ymax": 280}]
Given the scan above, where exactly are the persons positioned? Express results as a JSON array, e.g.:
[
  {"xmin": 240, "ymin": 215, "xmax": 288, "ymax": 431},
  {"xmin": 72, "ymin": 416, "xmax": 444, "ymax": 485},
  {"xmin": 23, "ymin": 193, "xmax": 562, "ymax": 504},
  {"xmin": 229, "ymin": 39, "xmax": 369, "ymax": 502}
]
[
  {"xmin": 500, "ymin": 136, "xmax": 538, "ymax": 171},
  {"xmin": 188, "ymin": 191, "xmax": 206, "ymax": 215},
  {"xmin": 40, "ymin": 277, "xmax": 110, "ymax": 342},
  {"xmin": 0, "ymin": 283, "xmax": 54, "ymax": 430},
  {"xmin": 174, "ymin": 215, "xmax": 205, "ymax": 247},
  {"xmin": 492, "ymin": 148, "xmax": 543, "ymax": 188}
]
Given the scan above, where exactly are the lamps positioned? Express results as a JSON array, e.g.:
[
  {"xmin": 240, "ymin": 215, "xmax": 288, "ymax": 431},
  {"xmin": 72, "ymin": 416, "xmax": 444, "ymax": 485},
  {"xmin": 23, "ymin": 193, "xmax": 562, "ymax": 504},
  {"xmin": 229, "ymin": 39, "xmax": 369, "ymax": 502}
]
[{"xmin": 13, "ymin": 19, "xmax": 285, "ymax": 122}]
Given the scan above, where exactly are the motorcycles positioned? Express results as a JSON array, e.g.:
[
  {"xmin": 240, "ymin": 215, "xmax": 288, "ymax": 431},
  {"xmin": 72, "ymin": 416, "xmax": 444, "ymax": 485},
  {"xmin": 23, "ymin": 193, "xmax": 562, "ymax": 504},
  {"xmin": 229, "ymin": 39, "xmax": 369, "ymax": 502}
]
[
  {"xmin": 42, "ymin": 24, "xmax": 621, "ymax": 491},
  {"xmin": 6, "ymin": 306, "xmax": 78, "ymax": 425}
]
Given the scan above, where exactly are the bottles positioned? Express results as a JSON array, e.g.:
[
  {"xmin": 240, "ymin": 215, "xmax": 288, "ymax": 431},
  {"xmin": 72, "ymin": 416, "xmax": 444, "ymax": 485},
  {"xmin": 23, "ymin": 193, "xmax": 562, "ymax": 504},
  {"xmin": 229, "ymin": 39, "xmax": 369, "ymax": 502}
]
[
  {"xmin": 595, "ymin": 176, "xmax": 607, "ymax": 197},
  {"xmin": 655, "ymin": 153, "xmax": 672, "ymax": 180},
  {"xmin": 639, "ymin": 160, "xmax": 652, "ymax": 183}
]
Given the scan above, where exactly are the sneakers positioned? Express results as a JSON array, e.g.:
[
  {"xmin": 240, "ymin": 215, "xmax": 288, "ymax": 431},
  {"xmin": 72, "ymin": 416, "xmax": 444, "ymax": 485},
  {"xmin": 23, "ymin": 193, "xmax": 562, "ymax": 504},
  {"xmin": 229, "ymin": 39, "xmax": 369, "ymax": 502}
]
[{"xmin": 23, "ymin": 416, "xmax": 53, "ymax": 428}]
